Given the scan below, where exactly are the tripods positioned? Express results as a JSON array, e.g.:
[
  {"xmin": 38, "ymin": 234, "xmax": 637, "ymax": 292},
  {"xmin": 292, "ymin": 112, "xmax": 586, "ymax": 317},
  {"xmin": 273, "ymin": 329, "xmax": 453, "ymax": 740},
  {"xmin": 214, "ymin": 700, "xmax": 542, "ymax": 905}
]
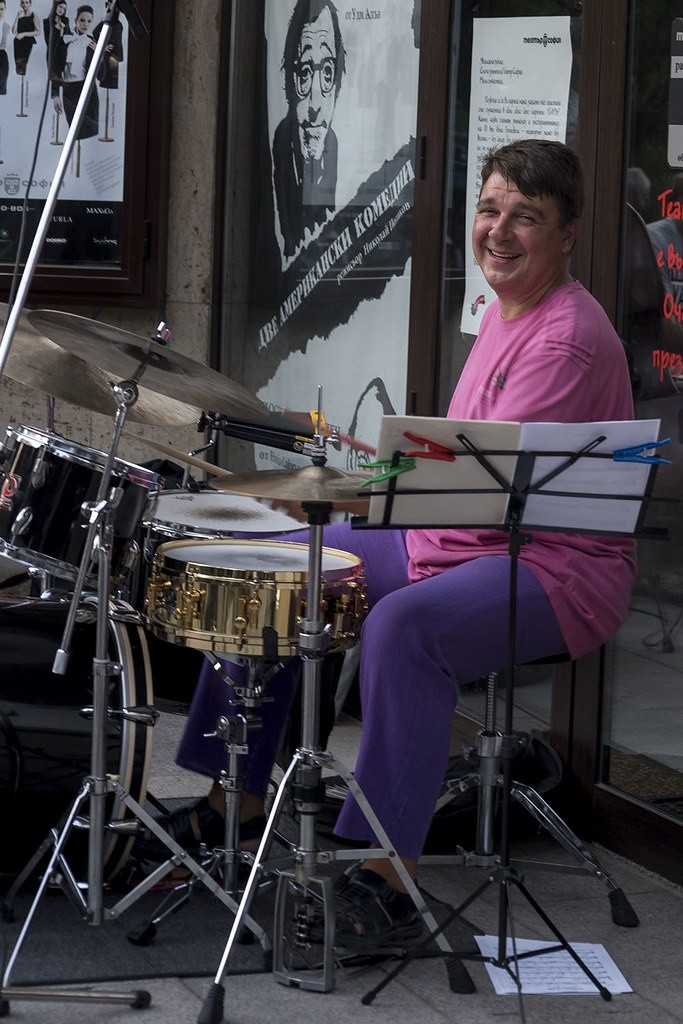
[{"xmin": 0, "ymin": 348, "xmax": 683, "ymax": 1024}]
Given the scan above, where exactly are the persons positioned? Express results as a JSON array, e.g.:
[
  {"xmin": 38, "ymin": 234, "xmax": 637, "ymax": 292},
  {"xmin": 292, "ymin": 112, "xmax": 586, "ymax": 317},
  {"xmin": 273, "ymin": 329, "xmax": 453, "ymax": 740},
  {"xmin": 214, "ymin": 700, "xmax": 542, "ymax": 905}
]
[
  {"xmin": 0, "ymin": 0, "xmax": 122, "ymax": 141},
  {"xmin": 129, "ymin": 140, "xmax": 633, "ymax": 946}
]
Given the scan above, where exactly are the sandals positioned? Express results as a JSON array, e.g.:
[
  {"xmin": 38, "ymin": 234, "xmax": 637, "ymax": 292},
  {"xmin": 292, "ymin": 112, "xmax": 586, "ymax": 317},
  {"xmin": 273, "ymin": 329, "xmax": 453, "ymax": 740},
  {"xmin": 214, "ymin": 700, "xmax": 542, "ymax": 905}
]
[
  {"xmin": 115, "ymin": 796, "xmax": 266, "ymax": 865},
  {"xmin": 286, "ymin": 869, "xmax": 426, "ymax": 946}
]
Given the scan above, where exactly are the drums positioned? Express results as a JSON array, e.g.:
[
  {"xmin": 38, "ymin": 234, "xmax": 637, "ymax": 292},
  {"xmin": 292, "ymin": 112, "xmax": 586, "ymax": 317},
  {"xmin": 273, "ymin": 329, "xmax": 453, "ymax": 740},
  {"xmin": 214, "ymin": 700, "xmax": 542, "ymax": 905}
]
[
  {"xmin": 143, "ymin": 486, "xmax": 310, "ymax": 539},
  {"xmin": 0, "ymin": 592, "xmax": 158, "ymax": 911},
  {"xmin": 0, "ymin": 424, "xmax": 168, "ymax": 592},
  {"xmin": 143, "ymin": 537, "xmax": 368, "ymax": 658}
]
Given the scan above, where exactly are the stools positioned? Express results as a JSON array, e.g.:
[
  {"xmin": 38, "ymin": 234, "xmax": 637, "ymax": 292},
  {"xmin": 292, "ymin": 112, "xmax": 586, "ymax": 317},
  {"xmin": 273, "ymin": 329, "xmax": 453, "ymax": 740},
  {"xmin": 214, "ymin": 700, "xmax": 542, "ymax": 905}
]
[{"xmin": 336, "ymin": 653, "xmax": 642, "ymax": 931}]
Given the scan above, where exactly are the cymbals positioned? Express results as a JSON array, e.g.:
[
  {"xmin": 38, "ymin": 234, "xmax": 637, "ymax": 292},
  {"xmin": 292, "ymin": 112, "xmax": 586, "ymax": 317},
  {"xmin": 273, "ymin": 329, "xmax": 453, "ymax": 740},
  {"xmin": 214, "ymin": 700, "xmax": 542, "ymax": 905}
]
[
  {"xmin": 0, "ymin": 296, "xmax": 205, "ymax": 426},
  {"xmin": 25, "ymin": 307, "xmax": 275, "ymax": 422},
  {"xmin": 209, "ymin": 465, "xmax": 376, "ymax": 502}
]
